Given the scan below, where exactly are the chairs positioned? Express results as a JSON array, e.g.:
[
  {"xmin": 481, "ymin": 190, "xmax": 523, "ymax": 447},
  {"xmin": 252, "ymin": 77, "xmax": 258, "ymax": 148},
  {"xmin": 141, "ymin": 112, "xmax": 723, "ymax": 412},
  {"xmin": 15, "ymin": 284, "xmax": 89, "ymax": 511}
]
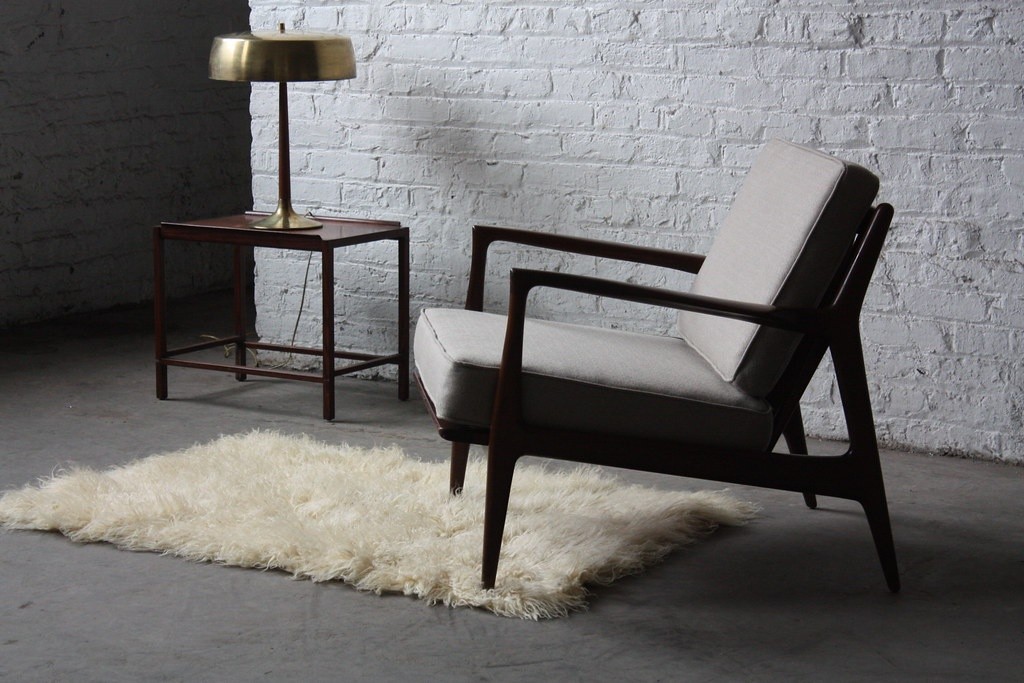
[{"xmin": 413, "ymin": 136, "xmax": 904, "ymax": 593}]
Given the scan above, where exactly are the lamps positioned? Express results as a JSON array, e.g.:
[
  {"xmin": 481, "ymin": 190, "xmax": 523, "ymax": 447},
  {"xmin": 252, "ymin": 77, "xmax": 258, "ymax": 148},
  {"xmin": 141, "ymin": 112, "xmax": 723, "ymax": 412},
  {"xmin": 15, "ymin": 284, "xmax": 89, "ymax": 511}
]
[{"xmin": 206, "ymin": 21, "xmax": 358, "ymax": 231}]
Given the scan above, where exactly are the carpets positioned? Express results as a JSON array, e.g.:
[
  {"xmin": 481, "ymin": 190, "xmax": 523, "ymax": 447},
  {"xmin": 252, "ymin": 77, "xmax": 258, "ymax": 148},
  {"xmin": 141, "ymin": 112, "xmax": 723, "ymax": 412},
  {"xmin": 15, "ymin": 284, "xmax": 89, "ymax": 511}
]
[{"xmin": 0, "ymin": 430, "xmax": 753, "ymax": 619}]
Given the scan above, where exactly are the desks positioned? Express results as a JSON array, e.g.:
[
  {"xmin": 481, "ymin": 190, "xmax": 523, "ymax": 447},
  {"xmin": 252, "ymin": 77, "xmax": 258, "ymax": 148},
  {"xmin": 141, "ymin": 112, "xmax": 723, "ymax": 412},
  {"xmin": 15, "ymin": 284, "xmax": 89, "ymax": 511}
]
[{"xmin": 151, "ymin": 208, "xmax": 412, "ymax": 417}]
